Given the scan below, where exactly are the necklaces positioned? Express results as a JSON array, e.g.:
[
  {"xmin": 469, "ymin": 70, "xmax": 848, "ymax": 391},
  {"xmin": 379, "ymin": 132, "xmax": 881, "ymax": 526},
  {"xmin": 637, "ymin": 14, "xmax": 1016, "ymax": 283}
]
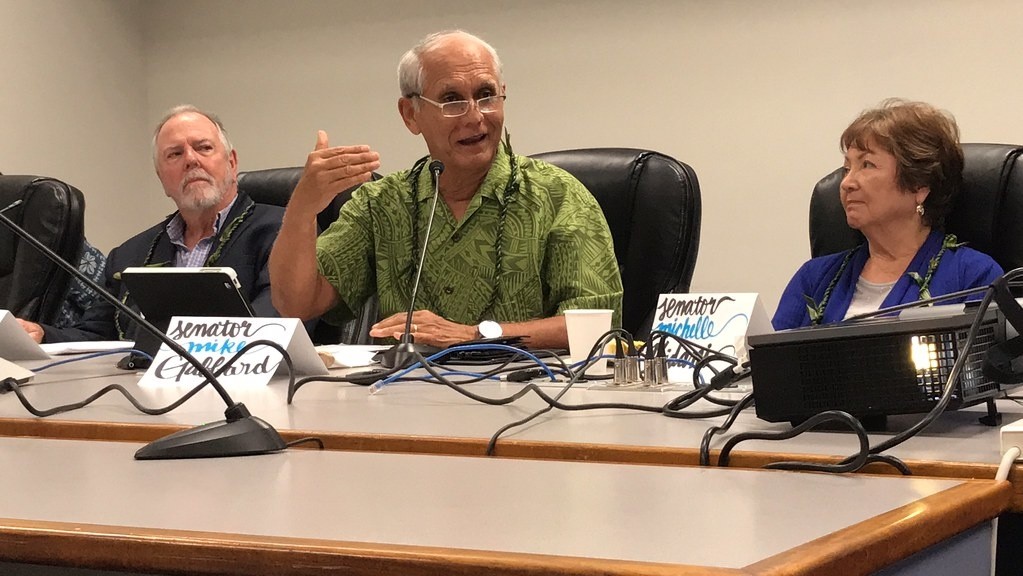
[
  {"xmin": 401, "ymin": 132, "xmax": 517, "ymax": 324},
  {"xmin": 800, "ymin": 231, "xmax": 969, "ymax": 324},
  {"xmin": 115, "ymin": 202, "xmax": 254, "ymax": 341}
]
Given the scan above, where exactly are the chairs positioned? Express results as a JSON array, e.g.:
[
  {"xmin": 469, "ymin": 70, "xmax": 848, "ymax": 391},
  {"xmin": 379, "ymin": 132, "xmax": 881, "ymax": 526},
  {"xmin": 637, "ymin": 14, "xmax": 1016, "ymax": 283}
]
[
  {"xmin": 525, "ymin": 148, "xmax": 703, "ymax": 337},
  {"xmin": 0, "ymin": 176, "xmax": 86, "ymax": 327},
  {"xmin": 809, "ymin": 143, "xmax": 1023, "ymax": 299},
  {"xmin": 236, "ymin": 166, "xmax": 385, "ymax": 346}
]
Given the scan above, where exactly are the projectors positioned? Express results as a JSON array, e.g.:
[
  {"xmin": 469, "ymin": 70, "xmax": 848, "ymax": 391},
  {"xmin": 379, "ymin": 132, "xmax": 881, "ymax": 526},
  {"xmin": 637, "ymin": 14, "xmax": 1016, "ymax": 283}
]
[{"xmin": 749, "ymin": 299, "xmax": 1023, "ymax": 434}]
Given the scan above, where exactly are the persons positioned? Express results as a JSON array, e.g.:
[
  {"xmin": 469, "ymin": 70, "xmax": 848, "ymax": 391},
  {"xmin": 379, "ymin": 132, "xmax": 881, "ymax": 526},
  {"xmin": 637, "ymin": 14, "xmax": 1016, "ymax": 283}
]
[
  {"xmin": 268, "ymin": 31, "xmax": 622, "ymax": 352},
  {"xmin": 104, "ymin": 104, "xmax": 323, "ymax": 341},
  {"xmin": 0, "ymin": 171, "xmax": 108, "ymax": 344},
  {"xmin": 770, "ymin": 96, "xmax": 1005, "ymax": 331}
]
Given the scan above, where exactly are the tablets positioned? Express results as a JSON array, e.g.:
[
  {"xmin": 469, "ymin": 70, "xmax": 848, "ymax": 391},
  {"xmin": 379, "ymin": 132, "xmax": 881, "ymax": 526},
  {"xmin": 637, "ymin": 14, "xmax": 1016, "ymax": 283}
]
[{"xmin": 122, "ymin": 266, "xmax": 254, "ymax": 320}]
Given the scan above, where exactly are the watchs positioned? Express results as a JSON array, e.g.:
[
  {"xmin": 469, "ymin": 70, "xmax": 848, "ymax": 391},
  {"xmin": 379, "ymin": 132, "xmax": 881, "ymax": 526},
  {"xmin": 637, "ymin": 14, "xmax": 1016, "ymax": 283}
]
[{"xmin": 475, "ymin": 319, "xmax": 502, "ymax": 339}]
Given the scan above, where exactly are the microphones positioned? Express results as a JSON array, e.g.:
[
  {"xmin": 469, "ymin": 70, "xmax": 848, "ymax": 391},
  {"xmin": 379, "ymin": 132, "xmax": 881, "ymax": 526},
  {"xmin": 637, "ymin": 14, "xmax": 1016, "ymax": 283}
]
[
  {"xmin": 372, "ymin": 158, "xmax": 450, "ymax": 369},
  {"xmin": 0, "ymin": 200, "xmax": 23, "ymax": 213}
]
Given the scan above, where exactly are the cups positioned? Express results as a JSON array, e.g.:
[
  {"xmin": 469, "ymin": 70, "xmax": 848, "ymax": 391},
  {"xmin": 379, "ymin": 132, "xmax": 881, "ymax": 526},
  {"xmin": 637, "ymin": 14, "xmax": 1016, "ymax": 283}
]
[{"xmin": 563, "ymin": 309, "xmax": 614, "ymax": 375}]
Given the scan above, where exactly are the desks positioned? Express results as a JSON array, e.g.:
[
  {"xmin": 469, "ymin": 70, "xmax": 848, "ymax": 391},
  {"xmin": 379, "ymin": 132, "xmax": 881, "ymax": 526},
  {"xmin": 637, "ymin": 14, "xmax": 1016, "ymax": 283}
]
[
  {"xmin": 0, "ymin": 355, "xmax": 1023, "ymax": 502},
  {"xmin": 0, "ymin": 432, "xmax": 1013, "ymax": 576}
]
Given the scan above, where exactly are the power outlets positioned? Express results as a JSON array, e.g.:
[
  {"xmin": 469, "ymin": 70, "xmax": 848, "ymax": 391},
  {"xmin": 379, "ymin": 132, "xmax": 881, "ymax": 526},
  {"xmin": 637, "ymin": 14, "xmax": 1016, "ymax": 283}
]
[{"xmin": 586, "ymin": 385, "xmax": 673, "ymax": 411}]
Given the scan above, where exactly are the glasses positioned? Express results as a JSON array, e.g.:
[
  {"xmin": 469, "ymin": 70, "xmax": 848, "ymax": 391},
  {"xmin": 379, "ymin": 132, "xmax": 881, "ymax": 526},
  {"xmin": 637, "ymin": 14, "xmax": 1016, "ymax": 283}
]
[{"xmin": 407, "ymin": 92, "xmax": 507, "ymax": 118}]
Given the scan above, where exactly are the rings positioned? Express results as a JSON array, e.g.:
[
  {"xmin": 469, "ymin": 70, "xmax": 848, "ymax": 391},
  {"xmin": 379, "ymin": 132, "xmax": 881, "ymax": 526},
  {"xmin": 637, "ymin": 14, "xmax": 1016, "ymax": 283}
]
[{"xmin": 413, "ymin": 325, "xmax": 417, "ymax": 332}]
